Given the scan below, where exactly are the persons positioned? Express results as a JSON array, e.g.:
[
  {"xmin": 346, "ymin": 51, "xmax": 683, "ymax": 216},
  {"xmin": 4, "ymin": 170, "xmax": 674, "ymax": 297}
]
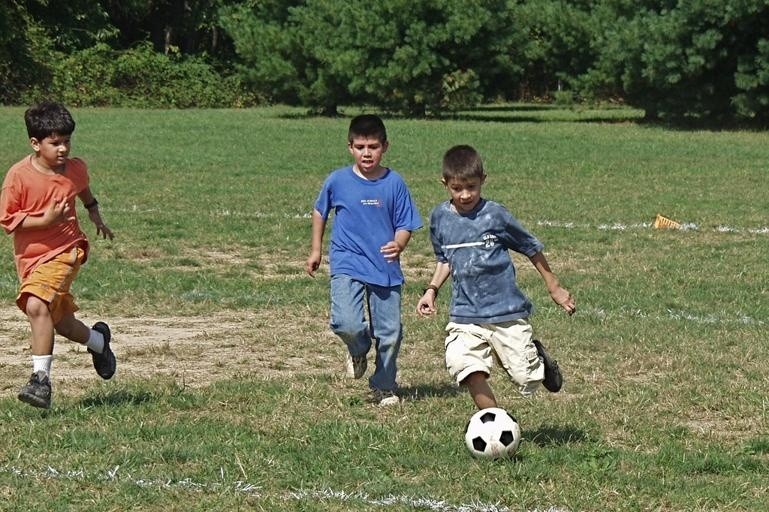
[
  {"xmin": 305, "ymin": 114, "xmax": 423, "ymax": 406},
  {"xmin": 415, "ymin": 145, "xmax": 576, "ymax": 410},
  {"xmin": 0, "ymin": 100, "xmax": 116, "ymax": 408}
]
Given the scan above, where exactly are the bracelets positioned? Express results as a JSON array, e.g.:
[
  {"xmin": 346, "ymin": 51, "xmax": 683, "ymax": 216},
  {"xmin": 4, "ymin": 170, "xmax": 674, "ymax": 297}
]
[{"xmin": 424, "ymin": 285, "xmax": 439, "ymax": 298}]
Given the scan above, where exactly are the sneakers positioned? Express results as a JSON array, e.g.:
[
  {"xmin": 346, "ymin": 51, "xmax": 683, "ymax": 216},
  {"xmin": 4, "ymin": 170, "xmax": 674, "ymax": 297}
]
[
  {"xmin": 86, "ymin": 323, "xmax": 115, "ymax": 380},
  {"xmin": 533, "ymin": 339, "xmax": 562, "ymax": 392},
  {"xmin": 369, "ymin": 382, "xmax": 399, "ymax": 406},
  {"xmin": 18, "ymin": 371, "xmax": 52, "ymax": 409},
  {"xmin": 346, "ymin": 341, "xmax": 372, "ymax": 379}
]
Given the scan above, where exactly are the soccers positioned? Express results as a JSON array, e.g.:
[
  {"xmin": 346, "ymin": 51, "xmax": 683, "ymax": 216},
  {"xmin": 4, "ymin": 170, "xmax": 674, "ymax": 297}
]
[{"xmin": 464, "ymin": 407, "xmax": 521, "ymax": 458}]
